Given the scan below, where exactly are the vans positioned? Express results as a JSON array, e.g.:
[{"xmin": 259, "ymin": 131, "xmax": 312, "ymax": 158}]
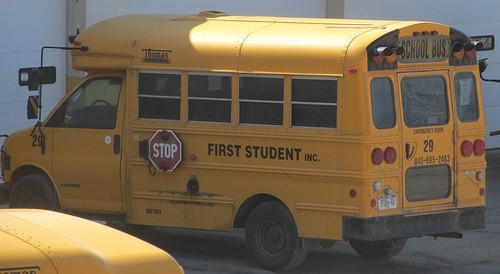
[{"xmin": 0, "ymin": 10, "xmax": 487, "ymax": 271}]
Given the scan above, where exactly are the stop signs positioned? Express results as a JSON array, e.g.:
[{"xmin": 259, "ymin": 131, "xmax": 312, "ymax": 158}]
[{"xmin": 148, "ymin": 129, "xmax": 181, "ymax": 172}]
